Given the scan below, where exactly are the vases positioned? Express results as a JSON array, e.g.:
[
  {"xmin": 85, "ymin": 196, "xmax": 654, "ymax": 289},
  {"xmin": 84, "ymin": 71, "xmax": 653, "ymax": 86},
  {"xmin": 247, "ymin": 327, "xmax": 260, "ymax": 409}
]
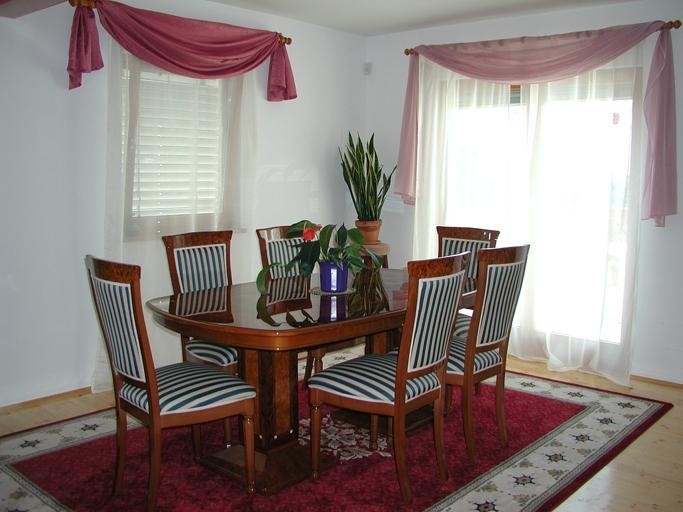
[
  {"xmin": 319, "ymin": 262, "xmax": 348, "ymax": 292},
  {"xmin": 312, "ymin": 294, "xmax": 347, "ymax": 323}
]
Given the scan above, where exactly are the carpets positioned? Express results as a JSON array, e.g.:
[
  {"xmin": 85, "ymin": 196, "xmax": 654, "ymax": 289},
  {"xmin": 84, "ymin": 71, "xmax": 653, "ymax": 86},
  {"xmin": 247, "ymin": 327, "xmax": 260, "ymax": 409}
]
[{"xmin": 0, "ymin": 371, "xmax": 676, "ymax": 511}]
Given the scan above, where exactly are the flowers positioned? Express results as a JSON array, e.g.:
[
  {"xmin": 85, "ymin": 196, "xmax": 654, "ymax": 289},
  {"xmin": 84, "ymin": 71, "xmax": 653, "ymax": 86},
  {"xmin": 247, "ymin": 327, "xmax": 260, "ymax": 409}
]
[{"xmin": 255, "ymin": 226, "xmax": 364, "ymax": 262}]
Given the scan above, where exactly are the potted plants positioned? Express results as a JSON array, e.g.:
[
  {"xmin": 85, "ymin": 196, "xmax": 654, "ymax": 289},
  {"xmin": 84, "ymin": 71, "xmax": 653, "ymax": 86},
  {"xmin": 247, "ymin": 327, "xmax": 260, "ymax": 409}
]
[{"xmin": 337, "ymin": 132, "xmax": 398, "ymax": 244}]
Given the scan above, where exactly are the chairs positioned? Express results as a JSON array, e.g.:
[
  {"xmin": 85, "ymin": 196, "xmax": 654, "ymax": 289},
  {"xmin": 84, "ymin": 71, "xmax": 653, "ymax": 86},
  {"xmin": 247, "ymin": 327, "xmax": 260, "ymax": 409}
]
[
  {"xmin": 387, "ymin": 243, "xmax": 532, "ymax": 456},
  {"xmin": 254, "ymin": 229, "xmax": 364, "ymax": 423},
  {"xmin": 161, "ymin": 232, "xmax": 281, "ymax": 449},
  {"xmin": 304, "ymin": 250, "xmax": 471, "ymax": 506},
  {"xmin": 433, "ymin": 227, "xmax": 502, "ymax": 398},
  {"xmin": 84, "ymin": 257, "xmax": 258, "ymax": 503}
]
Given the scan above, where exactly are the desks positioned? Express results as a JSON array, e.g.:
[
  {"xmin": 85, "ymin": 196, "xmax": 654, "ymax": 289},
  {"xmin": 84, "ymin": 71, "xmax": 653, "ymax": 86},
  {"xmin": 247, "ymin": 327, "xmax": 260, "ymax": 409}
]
[{"xmin": 143, "ymin": 267, "xmax": 459, "ymax": 496}]
